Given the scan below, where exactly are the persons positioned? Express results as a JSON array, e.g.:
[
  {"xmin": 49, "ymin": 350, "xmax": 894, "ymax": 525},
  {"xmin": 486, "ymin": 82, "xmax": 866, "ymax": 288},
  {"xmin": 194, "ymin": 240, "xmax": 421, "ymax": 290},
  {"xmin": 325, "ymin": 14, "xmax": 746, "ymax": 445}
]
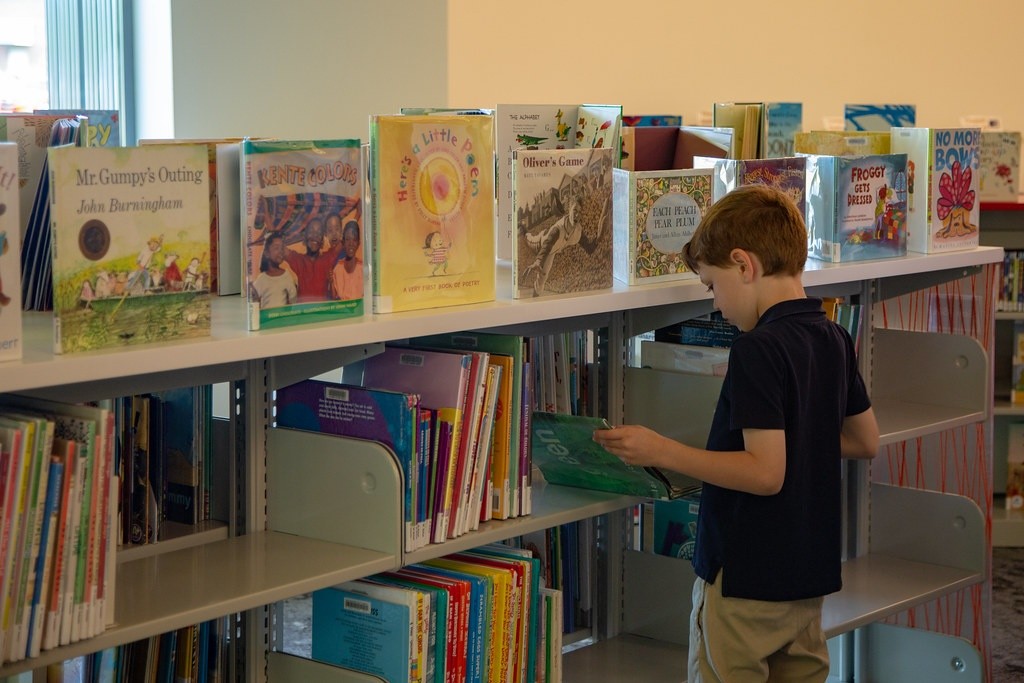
[{"xmin": 592, "ymin": 185, "xmax": 881, "ymax": 683}]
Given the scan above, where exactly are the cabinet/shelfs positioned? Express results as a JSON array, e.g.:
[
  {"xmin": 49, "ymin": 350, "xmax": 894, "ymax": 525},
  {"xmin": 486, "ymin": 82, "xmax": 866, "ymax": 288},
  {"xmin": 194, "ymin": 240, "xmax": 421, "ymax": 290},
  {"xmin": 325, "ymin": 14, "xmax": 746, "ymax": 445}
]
[
  {"xmin": 1, "ymin": 245, "xmax": 1006, "ymax": 683},
  {"xmin": 977, "ymin": 201, "xmax": 1024, "ymax": 549}
]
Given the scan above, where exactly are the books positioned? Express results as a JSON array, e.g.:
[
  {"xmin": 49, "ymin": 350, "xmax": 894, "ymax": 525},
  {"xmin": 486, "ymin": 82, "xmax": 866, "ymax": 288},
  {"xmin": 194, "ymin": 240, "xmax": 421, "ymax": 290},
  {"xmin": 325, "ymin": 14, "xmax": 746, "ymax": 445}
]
[{"xmin": 0, "ymin": 100, "xmax": 1024, "ymax": 683}]
